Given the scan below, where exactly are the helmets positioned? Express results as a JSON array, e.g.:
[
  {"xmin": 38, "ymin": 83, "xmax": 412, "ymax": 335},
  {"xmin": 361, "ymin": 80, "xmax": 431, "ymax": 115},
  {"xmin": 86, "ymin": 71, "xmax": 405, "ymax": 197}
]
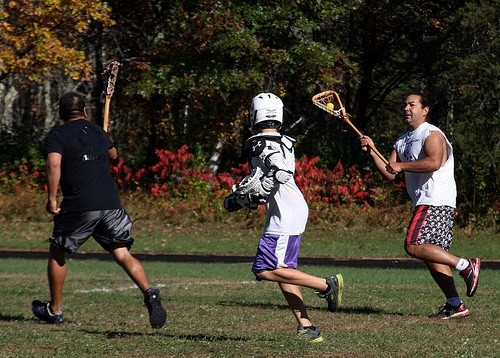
[{"xmin": 251, "ymin": 93, "xmax": 284, "ymax": 133}]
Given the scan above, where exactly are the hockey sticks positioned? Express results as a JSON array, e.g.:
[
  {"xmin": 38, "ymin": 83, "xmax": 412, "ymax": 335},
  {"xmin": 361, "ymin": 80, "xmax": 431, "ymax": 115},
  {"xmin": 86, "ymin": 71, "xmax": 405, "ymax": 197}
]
[
  {"xmin": 101, "ymin": 62, "xmax": 119, "ymax": 133},
  {"xmin": 312, "ymin": 91, "xmax": 399, "ymax": 174}
]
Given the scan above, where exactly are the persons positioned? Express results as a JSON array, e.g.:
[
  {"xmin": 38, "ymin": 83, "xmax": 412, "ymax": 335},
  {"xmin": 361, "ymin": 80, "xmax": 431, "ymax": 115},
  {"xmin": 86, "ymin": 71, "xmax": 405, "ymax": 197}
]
[
  {"xmin": 32, "ymin": 91, "xmax": 167, "ymax": 329},
  {"xmin": 360, "ymin": 91, "xmax": 481, "ymax": 320},
  {"xmin": 223, "ymin": 92, "xmax": 343, "ymax": 344}
]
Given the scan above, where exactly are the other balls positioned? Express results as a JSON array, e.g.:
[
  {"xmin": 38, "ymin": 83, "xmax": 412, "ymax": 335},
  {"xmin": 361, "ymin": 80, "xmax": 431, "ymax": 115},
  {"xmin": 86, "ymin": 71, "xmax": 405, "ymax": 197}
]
[{"xmin": 326, "ymin": 103, "xmax": 334, "ymax": 109}]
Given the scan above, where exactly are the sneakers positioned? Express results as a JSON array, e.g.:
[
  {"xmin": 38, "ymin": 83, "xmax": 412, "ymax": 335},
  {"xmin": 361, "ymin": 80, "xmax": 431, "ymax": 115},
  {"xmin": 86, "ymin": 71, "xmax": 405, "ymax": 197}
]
[
  {"xmin": 428, "ymin": 301, "xmax": 470, "ymax": 320},
  {"xmin": 459, "ymin": 257, "xmax": 481, "ymax": 297},
  {"xmin": 32, "ymin": 300, "xmax": 64, "ymax": 324},
  {"xmin": 294, "ymin": 324, "xmax": 323, "ymax": 344},
  {"xmin": 144, "ymin": 287, "xmax": 166, "ymax": 330},
  {"xmin": 314, "ymin": 273, "xmax": 343, "ymax": 312}
]
